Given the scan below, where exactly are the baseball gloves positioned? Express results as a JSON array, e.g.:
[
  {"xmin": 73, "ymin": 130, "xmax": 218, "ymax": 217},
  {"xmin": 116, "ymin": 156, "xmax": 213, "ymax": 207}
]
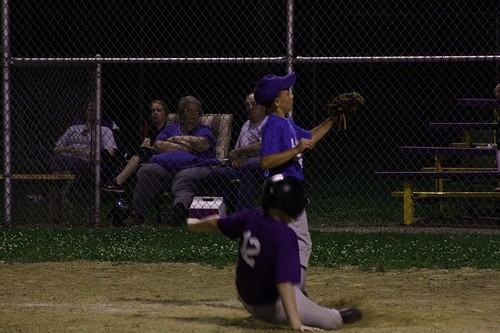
[{"xmin": 326, "ymin": 91, "xmax": 366, "ymax": 133}]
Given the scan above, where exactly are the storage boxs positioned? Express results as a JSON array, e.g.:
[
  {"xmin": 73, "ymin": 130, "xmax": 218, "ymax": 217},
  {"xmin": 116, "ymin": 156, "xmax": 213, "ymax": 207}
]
[{"xmin": 189, "ymin": 196, "xmax": 226, "ymax": 219}]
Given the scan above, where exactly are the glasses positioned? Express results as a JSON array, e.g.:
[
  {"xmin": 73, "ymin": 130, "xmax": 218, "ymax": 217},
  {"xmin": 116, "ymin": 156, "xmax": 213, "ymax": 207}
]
[{"xmin": 183, "ymin": 108, "xmax": 200, "ymax": 113}]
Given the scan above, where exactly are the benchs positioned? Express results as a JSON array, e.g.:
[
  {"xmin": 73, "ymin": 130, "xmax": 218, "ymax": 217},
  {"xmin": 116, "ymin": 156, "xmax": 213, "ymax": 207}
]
[
  {"xmin": 375, "ymin": 99, "xmax": 500, "ymax": 226},
  {"xmin": 0, "ymin": 173, "xmax": 77, "ymax": 225}
]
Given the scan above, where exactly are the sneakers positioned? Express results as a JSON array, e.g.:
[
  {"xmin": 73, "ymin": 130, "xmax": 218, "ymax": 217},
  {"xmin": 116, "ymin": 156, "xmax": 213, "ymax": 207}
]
[{"xmin": 100, "ymin": 177, "xmax": 124, "ymax": 193}]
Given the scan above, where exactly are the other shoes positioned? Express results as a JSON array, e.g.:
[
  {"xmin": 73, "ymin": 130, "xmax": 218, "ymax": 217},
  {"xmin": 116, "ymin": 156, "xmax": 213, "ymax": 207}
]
[
  {"xmin": 122, "ymin": 213, "xmax": 140, "ymax": 227},
  {"xmin": 341, "ymin": 306, "xmax": 362, "ymax": 326}
]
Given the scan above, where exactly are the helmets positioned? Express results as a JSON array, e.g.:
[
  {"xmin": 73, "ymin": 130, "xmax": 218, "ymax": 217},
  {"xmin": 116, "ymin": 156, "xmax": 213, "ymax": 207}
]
[{"xmin": 261, "ymin": 172, "xmax": 306, "ymax": 218}]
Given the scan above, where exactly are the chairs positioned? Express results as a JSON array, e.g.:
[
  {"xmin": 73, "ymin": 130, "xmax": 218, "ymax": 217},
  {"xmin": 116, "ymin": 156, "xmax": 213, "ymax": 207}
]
[{"xmin": 152, "ymin": 114, "xmax": 233, "ymax": 225}]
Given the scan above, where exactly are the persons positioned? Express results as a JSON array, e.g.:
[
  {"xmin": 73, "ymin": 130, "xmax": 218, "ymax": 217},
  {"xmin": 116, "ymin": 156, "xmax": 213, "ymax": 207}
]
[
  {"xmin": 184, "ymin": 172, "xmax": 363, "ymax": 332},
  {"xmin": 253, "ymin": 72, "xmax": 339, "ymax": 298},
  {"xmin": 100, "ymin": 92, "xmax": 270, "ymax": 228},
  {"xmin": 0, "ymin": 99, "xmax": 119, "ymax": 227}
]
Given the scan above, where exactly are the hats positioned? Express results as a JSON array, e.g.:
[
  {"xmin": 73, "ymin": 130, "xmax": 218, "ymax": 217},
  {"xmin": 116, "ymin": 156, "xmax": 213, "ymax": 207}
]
[{"xmin": 254, "ymin": 73, "xmax": 296, "ymax": 106}]
